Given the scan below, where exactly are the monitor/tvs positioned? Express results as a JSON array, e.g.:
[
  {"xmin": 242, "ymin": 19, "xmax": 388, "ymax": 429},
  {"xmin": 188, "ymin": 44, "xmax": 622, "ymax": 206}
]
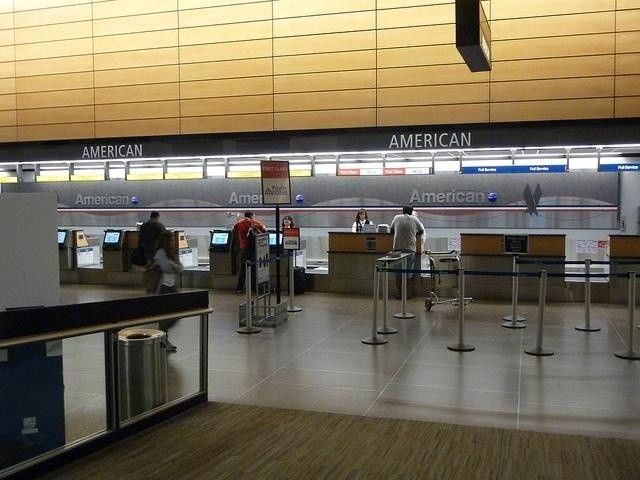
[
  {"xmin": 58, "ymin": 229, "xmax": 68, "ymax": 245},
  {"xmin": 103, "ymin": 229, "xmax": 122, "ymax": 246},
  {"xmin": 268, "ymin": 230, "xmax": 283, "ymax": 246},
  {"xmin": 210, "ymin": 230, "xmax": 230, "ymax": 247}
]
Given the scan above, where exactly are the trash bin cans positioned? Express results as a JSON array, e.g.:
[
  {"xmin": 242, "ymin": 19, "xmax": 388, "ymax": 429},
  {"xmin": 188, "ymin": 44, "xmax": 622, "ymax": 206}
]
[{"xmin": 117, "ymin": 328, "xmax": 167, "ymax": 422}]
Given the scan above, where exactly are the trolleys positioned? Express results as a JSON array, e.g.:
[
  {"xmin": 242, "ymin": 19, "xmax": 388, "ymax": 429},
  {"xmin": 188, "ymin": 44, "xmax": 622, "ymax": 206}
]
[{"xmin": 425, "ymin": 250, "xmax": 474, "ymax": 311}]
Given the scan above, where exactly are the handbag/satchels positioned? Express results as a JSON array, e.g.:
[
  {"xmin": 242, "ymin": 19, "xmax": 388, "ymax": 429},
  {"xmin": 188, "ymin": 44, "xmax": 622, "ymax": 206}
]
[
  {"xmin": 143, "ymin": 259, "xmax": 159, "ymax": 291},
  {"xmin": 246, "ymin": 225, "xmax": 262, "ymax": 241},
  {"xmin": 130, "ymin": 247, "xmax": 146, "ymax": 265}
]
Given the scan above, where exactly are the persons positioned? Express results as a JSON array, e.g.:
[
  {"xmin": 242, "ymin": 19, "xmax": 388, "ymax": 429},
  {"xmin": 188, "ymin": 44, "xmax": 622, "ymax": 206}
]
[
  {"xmin": 351, "ymin": 209, "xmax": 374, "ymax": 233},
  {"xmin": 232, "ymin": 210, "xmax": 271, "ymax": 294},
  {"xmin": 390, "ymin": 204, "xmax": 426, "ymax": 300},
  {"xmin": 280, "ymin": 216, "xmax": 295, "ymax": 229},
  {"xmin": 151, "ymin": 230, "xmax": 184, "ymax": 352},
  {"xmin": 137, "ymin": 210, "xmax": 167, "ymax": 293}
]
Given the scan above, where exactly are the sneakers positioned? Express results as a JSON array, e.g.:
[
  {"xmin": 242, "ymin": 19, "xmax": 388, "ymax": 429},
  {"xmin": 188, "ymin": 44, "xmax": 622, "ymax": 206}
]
[{"xmin": 166, "ymin": 339, "xmax": 177, "ymax": 351}]
